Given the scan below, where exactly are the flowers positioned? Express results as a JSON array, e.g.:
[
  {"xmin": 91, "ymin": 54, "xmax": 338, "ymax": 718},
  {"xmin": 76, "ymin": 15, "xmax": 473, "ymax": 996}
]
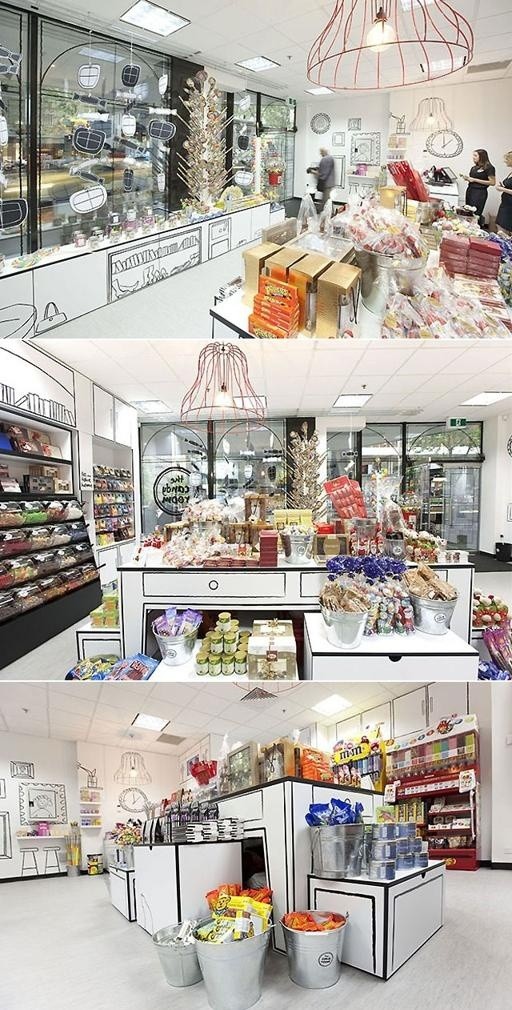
[{"xmin": 265, "ymin": 160, "xmax": 287, "ymax": 174}]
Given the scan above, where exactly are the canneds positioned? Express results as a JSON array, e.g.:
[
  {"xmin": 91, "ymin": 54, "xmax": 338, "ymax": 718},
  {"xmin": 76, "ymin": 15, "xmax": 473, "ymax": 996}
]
[
  {"xmin": 363, "ymin": 821, "xmax": 430, "ymax": 882},
  {"xmin": 194, "ymin": 612, "xmax": 249, "ymax": 676}
]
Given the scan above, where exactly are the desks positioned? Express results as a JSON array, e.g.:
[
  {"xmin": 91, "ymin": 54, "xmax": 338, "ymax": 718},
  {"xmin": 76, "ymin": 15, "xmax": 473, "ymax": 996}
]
[{"xmin": 209, "ymin": 238, "xmax": 511, "ymax": 338}]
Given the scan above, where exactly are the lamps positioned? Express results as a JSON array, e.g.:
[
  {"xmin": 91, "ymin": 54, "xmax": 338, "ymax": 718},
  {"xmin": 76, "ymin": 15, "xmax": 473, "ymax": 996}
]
[
  {"xmin": 178, "ymin": 341, "xmax": 266, "ymax": 435},
  {"xmin": 306, "ymin": 0, "xmax": 474, "ymax": 90}
]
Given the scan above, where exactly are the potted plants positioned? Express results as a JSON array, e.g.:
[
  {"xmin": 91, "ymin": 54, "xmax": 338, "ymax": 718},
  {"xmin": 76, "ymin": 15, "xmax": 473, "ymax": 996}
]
[{"xmin": 70, "ymin": 821, "xmax": 78, "ymax": 834}]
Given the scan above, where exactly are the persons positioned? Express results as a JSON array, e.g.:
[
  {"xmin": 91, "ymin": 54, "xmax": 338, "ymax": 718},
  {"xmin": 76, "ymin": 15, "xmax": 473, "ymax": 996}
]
[
  {"xmin": 458, "ymin": 147, "xmax": 497, "ymax": 224},
  {"xmin": 495, "ymin": 150, "xmax": 511, "ymax": 237},
  {"xmin": 307, "ymin": 148, "xmax": 336, "ymax": 211}
]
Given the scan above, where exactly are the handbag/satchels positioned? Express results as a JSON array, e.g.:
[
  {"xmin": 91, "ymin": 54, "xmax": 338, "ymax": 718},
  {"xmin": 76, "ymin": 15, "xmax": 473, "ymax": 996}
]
[{"xmin": 317, "ymin": 180, "xmax": 326, "ymax": 192}]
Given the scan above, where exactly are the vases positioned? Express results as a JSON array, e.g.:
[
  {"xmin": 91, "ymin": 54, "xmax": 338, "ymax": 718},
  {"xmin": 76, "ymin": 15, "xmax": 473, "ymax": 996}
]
[{"xmin": 268, "ymin": 170, "xmax": 283, "ymax": 186}]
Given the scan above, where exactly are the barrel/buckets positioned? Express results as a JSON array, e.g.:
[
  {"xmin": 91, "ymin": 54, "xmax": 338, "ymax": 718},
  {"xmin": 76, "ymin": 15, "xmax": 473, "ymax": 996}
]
[
  {"xmin": 310, "ymin": 823, "xmax": 365, "ymax": 879},
  {"xmin": 318, "ymin": 599, "xmax": 369, "ymax": 649},
  {"xmin": 279, "ymin": 530, "xmax": 314, "ymax": 564},
  {"xmin": 153, "ymin": 621, "xmax": 201, "ymax": 667},
  {"xmin": 351, "ymin": 241, "xmax": 429, "ymax": 318},
  {"xmin": 407, "ymin": 589, "xmax": 457, "ymax": 635}
]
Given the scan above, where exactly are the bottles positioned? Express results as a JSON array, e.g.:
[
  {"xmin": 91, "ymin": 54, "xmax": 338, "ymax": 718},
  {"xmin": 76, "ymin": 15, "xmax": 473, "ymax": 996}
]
[
  {"xmin": 384, "ymin": 531, "xmax": 405, "ymax": 559},
  {"xmin": 195, "ymin": 611, "xmax": 251, "ymax": 674}
]
